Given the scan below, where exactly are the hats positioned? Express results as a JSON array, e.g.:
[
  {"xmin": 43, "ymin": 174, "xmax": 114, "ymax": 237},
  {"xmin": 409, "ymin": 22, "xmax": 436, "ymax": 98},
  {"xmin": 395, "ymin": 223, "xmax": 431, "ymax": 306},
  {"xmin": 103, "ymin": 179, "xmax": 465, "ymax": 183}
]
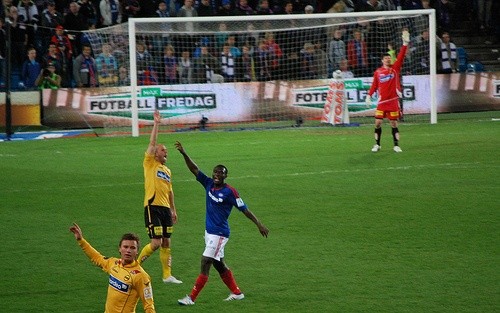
[{"xmin": 56, "ymin": 26, "xmax": 64, "ymax": 30}]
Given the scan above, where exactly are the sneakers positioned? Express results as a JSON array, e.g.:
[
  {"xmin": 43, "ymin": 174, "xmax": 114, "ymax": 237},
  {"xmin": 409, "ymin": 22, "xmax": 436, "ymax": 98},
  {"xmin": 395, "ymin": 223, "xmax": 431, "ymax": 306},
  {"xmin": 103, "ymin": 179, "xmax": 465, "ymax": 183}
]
[
  {"xmin": 162, "ymin": 276, "xmax": 183, "ymax": 284},
  {"xmin": 223, "ymin": 293, "xmax": 245, "ymax": 301},
  {"xmin": 177, "ymin": 294, "xmax": 195, "ymax": 306}
]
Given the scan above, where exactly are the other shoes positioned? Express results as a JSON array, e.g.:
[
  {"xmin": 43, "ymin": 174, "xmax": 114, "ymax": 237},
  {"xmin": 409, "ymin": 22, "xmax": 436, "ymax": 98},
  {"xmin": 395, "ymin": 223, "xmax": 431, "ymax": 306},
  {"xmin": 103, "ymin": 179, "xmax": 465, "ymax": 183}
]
[
  {"xmin": 402, "ymin": 30, "xmax": 410, "ymax": 44},
  {"xmin": 371, "ymin": 144, "xmax": 381, "ymax": 152},
  {"xmin": 365, "ymin": 94, "xmax": 372, "ymax": 107},
  {"xmin": 393, "ymin": 146, "xmax": 402, "ymax": 153}
]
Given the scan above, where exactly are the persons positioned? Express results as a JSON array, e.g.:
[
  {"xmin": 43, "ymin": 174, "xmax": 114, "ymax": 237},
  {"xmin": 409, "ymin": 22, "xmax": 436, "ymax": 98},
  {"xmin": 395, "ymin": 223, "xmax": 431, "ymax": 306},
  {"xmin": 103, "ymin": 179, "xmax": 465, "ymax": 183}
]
[
  {"xmin": 364, "ymin": 32, "xmax": 409, "ymax": 153},
  {"xmin": 175, "ymin": 141, "xmax": 269, "ymax": 305},
  {"xmin": 69, "ymin": 222, "xmax": 156, "ymax": 313},
  {"xmin": 137, "ymin": 109, "xmax": 183, "ymax": 284},
  {"xmin": 0, "ymin": 0, "xmax": 492, "ymax": 91}
]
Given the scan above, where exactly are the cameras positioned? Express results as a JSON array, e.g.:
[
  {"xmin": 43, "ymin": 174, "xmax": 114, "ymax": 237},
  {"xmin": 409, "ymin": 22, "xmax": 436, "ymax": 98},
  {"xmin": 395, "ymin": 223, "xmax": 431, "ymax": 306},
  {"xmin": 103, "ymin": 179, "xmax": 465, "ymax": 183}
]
[{"xmin": 43, "ymin": 70, "xmax": 51, "ymax": 76}]
